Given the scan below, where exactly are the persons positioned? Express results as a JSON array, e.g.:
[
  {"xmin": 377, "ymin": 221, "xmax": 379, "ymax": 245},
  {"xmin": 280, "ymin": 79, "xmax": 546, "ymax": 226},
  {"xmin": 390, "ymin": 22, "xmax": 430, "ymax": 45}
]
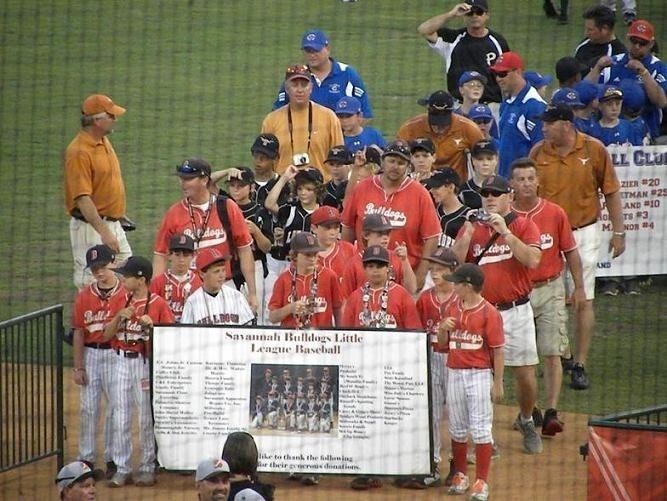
[
  {"xmin": 267, "ymin": 231, "xmax": 344, "ymax": 487},
  {"xmin": 487, "ymin": 52, "xmax": 552, "ymax": 178},
  {"xmin": 260, "ymin": 63, "xmax": 344, "ymax": 187},
  {"xmin": 505, "ymin": 158, "xmax": 588, "ymax": 437},
  {"xmin": 522, "ymin": 72, "xmax": 552, "ymax": 100},
  {"xmin": 583, "ymin": 21, "xmax": 667, "ymax": 138},
  {"xmin": 308, "ymin": 204, "xmax": 368, "ymax": 313},
  {"xmin": 294, "ymin": 375, "xmax": 305, "ymax": 394},
  {"xmin": 340, "ymin": 244, "xmax": 427, "ymax": 490},
  {"xmin": 249, "ymin": 132, "xmax": 293, "ymax": 279},
  {"xmin": 408, "ymin": 136, "xmax": 436, "ymax": 186},
  {"xmin": 321, "ymin": 143, "xmax": 356, "ymax": 239},
  {"xmin": 272, "ymin": 30, "xmax": 373, "ymax": 128},
  {"xmin": 208, "ymin": 166, "xmax": 273, "ymax": 327},
  {"xmin": 318, "ymin": 389, "xmax": 332, "ymax": 432},
  {"xmin": 571, "ymin": 5, "xmax": 632, "ymax": 80},
  {"xmin": 437, "ymin": 261, "xmax": 505, "ymax": 501},
  {"xmin": 63, "ymin": 94, "xmax": 135, "ymax": 346},
  {"xmin": 394, "ymin": 89, "xmax": 486, "ymax": 185},
  {"xmin": 265, "ymin": 391, "xmax": 278, "ymax": 429},
  {"xmin": 417, "ymin": 242, "xmax": 460, "ymax": 488},
  {"xmin": 295, "ymin": 391, "xmax": 307, "ymax": 432},
  {"xmin": 249, "ymin": 395, "xmax": 265, "ymax": 431},
  {"xmin": 451, "ymin": 174, "xmax": 543, "ymax": 459},
  {"xmin": 222, "ymin": 433, "xmax": 275, "ymax": 501},
  {"xmin": 319, "ymin": 377, "xmax": 330, "ymax": 394},
  {"xmin": 195, "ymin": 456, "xmax": 236, "ymax": 501},
  {"xmin": 149, "ymin": 158, "xmax": 258, "ymax": 320},
  {"xmin": 71, "ymin": 244, "xmax": 130, "ymax": 480},
  {"xmin": 588, "ymin": 84, "xmax": 642, "ymax": 146},
  {"xmin": 54, "ymin": 461, "xmax": 105, "ymax": 501},
  {"xmin": 601, "ymin": 0, "xmax": 637, "ymax": 26},
  {"xmin": 452, "ymin": 70, "xmax": 499, "ymax": 140},
  {"xmin": 417, "ymin": 0, "xmax": 515, "ymax": 140},
  {"xmin": 180, "ymin": 248, "xmax": 256, "ymax": 328},
  {"xmin": 617, "ymin": 78, "xmax": 654, "ymax": 147},
  {"xmin": 268, "ymin": 375, "xmax": 280, "ymax": 396},
  {"xmin": 550, "ymin": 86, "xmax": 584, "ymax": 122},
  {"xmin": 332, "ymin": 96, "xmax": 388, "ymax": 153},
  {"xmin": 260, "ymin": 368, "xmax": 272, "ymax": 395},
  {"xmin": 419, "ymin": 166, "xmax": 473, "ymax": 253},
  {"xmin": 306, "ymin": 367, "xmax": 314, "ymax": 378},
  {"xmin": 281, "ymin": 368, "xmax": 291, "ymax": 378},
  {"xmin": 543, "ymin": 0, "xmax": 568, "ymax": 25},
  {"xmin": 262, "ymin": 164, "xmax": 327, "ymax": 327},
  {"xmin": 573, "ymin": 79, "xmax": 601, "ymax": 138},
  {"xmin": 102, "ymin": 256, "xmax": 175, "ymax": 487},
  {"xmin": 459, "ymin": 138, "xmax": 514, "ymax": 210},
  {"xmin": 306, "ymin": 391, "xmax": 318, "ymax": 431},
  {"xmin": 359, "ymin": 146, "xmax": 384, "ymax": 184},
  {"xmin": 556, "ymin": 56, "xmax": 588, "ymax": 88},
  {"xmin": 467, "ymin": 104, "xmax": 500, "ymax": 153},
  {"xmin": 320, "ymin": 365, "xmax": 331, "ymax": 377},
  {"xmin": 529, "ymin": 103, "xmax": 626, "ymax": 389},
  {"xmin": 281, "ymin": 376, "xmax": 293, "ymax": 396},
  {"xmin": 306, "ymin": 376, "xmax": 317, "ymax": 396},
  {"xmin": 279, "ymin": 390, "xmax": 296, "ymax": 430},
  {"xmin": 340, "ymin": 142, "xmax": 443, "ymax": 293},
  {"xmin": 341, "ymin": 212, "xmax": 421, "ymax": 298}
]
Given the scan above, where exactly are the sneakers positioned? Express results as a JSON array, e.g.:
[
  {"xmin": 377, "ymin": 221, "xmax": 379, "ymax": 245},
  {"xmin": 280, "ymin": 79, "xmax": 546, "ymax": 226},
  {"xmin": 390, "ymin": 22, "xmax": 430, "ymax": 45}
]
[
  {"xmin": 105, "ymin": 470, "xmax": 133, "ymax": 486},
  {"xmin": 135, "ymin": 473, "xmax": 154, "ymax": 485},
  {"xmin": 444, "ymin": 472, "xmax": 467, "ymax": 490},
  {"xmin": 466, "ymin": 441, "xmax": 499, "ymax": 465},
  {"xmin": 519, "ymin": 414, "xmax": 546, "ymax": 457},
  {"xmin": 350, "ymin": 476, "xmax": 384, "ymax": 492},
  {"xmin": 559, "ymin": 11, "xmax": 569, "ymax": 24},
  {"xmin": 299, "ymin": 474, "xmax": 320, "ymax": 487},
  {"xmin": 623, "ymin": 14, "xmax": 635, "ymax": 26},
  {"xmin": 542, "ymin": 406, "xmax": 561, "ymax": 437},
  {"xmin": 407, "ymin": 467, "xmax": 438, "ymax": 488},
  {"xmin": 568, "ymin": 359, "xmax": 596, "ymax": 394},
  {"xmin": 471, "ymin": 480, "xmax": 488, "ymax": 499},
  {"xmin": 626, "ymin": 276, "xmax": 641, "ymax": 292},
  {"xmin": 546, "ymin": 11, "xmax": 558, "ymax": 22},
  {"xmin": 512, "ymin": 406, "xmax": 542, "ymax": 432},
  {"xmin": 543, "ymin": 351, "xmax": 570, "ymax": 376},
  {"xmin": 286, "ymin": 472, "xmax": 303, "ymax": 479},
  {"xmin": 604, "ymin": 282, "xmax": 619, "ymax": 297}
]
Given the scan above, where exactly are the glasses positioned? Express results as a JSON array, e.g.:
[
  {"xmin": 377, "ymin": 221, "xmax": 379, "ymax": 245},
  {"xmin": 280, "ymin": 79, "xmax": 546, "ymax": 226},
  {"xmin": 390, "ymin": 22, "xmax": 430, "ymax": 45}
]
[
  {"xmin": 479, "ymin": 189, "xmax": 511, "ymax": 201},
  {"xmin": 458, "ymin": 80, "xmax": 485, "ymax": 88},
  {"xmin": 494, "ymin": 71, "xmax": 508, "ymax": 78},
  {"xmin": 461, "ymin": 9, "xmax": 485, "ymax": 16},
  {"xmin": 474, "ymin": 117, "xmax": 490, "ymax": 124},
  {"xmin": 630, "ymin": 37, "xmax": 649, "ymax": 46}
]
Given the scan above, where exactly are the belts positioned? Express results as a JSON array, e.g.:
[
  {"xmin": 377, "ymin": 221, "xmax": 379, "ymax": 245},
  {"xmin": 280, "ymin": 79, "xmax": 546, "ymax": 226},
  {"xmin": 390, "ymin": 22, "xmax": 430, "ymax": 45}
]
[
  {"xmin": 114, "ymin": 349, "xmax": 146, "ymax": 360},
  {"xmin": 527, "ymin": 272, "xmax": 563, "ymax": 287},
  {"xmin": 71, "ymin": 206, "xmax": 128, "ymax": 225},
  {"xmin": 563, "ymin": 218, "xmax": 599, "ymax": 236},
  {"xmin": 80, "ymin": 339, "xmax": 117, "ymax": 353},
  {"xmin": 487, "ymin": 296, "xmax": 531, "ymax": 311}
]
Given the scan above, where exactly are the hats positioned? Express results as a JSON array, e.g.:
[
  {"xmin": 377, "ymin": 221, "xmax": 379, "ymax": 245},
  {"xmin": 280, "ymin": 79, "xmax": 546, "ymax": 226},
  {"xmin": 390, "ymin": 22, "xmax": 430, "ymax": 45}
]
[
  {"xmin": 321, "ymin": 144, "xmax": 355, "ymax": 165},
  {"xmin": 309, "ymin": 205, "xmax": 345, "ymax": 225},
  {"xmin": 419, "ymin": 245, "xmax": 461, "ymax": 269},
  {"xmin": 545, "ymin": 84, "xmax": 587, "ymax": 108},
  {"xmin": 617, "ymin": 77, "xmax": 646, "ymax": 114},
  {"xmin": 458, "ymin": 0, "xmax": 489, "ymax": 15},
  {"xmin": 107, "ymin": 255, "xmax": 154, "ymax": 281},
  {"xmin": 333, "ymin": 96, "xmax": 362, "ymax": 116},
  {"xmin": 380, "ymin": 138, "xmax": 413, "ymax": 163},
  {"xmin": 285, "ymin": 62, "xmax": 312, "ymax": 83},
  {"xmin": 443, "ymin": 261, "xmax": 486, "ymax": 285},
  {"xmin": 423, "ymin": 90, "xmax": 456, "ymax": 127},
  {"xmin": 595, "ymin": 83, "xmax": 624, "ymax": 103},
  {"xmin": 224, "ymin": 166, "xmax": 256, "ymax": 186},
  {"xmin": 173, "ymin": 156, "xmax": 213, "ymax": 179},
  {"xmin": 488, "ymin": 50, "xmax": 526, "ymax": 74},
  {"xmin": 288, "ymin": 231, "xmax": 328, "ymax": 253},
  {"xmin": 419, "ymin": 165, "xmax": 461, "ymax": 195},
  {"xmin": 361, "ymin": 212, "xmax": 399, "ymax": 233},
  {"xmin": 625, "ymin": 18, "xmax": 655, "ymax": 41},
  {"xmin": 251, "ymin": 131, "xmax": 280, "ymax": 159},
  {"xmin": 196, "ymin": 247, "xmax": 233, "ymax": 271},
  {"xmin": 81, "ymin": 94, "xmax": 127, "ymax": 117},
  {"xmin": 79, "ymin": 244, "xmax": 115, "ymax": 270},
  {"xmin": 409, "ymin": 135, "xmax": 436, "ymax": 154},
  {"xmin": 300, "ymin": 27, "xmax": 328, "ymax": 53},
  {"xmin": 519, "ymin": 67, "xmax": 550, "ymax": 92},
  {"xmin": 169, "ymin": 234, "xmax": 194, "ymax": 252},
  {"xmin": 193, "ymin": 458, "xmax": 235, "ymax": 484},
  {"xmin": 456, "ymin": 71, "xmax": 488, "ymax": 83},
  {"xmin": 470, "ymin": 137, "xmax": 502, "ymax": 156},
  {"xmin": 573, "ymin": 79, "xmax": 599, "ymax": 108},
  {"xmin": 292, "ymin": 166, "xmax": 325, "ymax": 183},
  {"xmin": 53, "ymin": 460, "xmax": 109, "ymax": 492},
  {"xmin": 360, "ymin": 244, "xmax": 391, "ymax": 265},
  {"xmin": 552, "ymin": 53, "xmax": 589, "ymax": 83},
  {"xmin": 355, "ymin": 142, "xmax": 380, "ymax": 169},
  {"xmin": 468, "ymin": 102, "xmax": 493, "ymax": 121},
  {"xmin": 532, "ymin": 105, "xmax": 576, "ymax": 123}
]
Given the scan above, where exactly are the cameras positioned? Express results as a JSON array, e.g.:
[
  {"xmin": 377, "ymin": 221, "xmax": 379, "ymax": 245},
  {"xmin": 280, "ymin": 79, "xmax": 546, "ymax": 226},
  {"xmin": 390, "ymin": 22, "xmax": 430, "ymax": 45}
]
[
  {"xmin": 293, "ymin": 153, "xmax": 309, "ymax": 166},
  {"xmin": 468, "ymin": 208, "xmax": 489, "ymax": 222}
]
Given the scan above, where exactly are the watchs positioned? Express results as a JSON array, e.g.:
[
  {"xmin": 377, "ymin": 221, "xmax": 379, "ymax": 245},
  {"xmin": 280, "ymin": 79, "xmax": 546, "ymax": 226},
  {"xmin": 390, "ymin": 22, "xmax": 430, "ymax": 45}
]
[
  {"xmin": 72, "ymin": 367, "xmax": 86, "ymax": 372},
  {"xmin": 501, "ymin": 229, "xmax": 512, "ymax": 238},
  {"xmin": 611, "ymin": 231, "xmax": 627, "ymax": 237}
]
